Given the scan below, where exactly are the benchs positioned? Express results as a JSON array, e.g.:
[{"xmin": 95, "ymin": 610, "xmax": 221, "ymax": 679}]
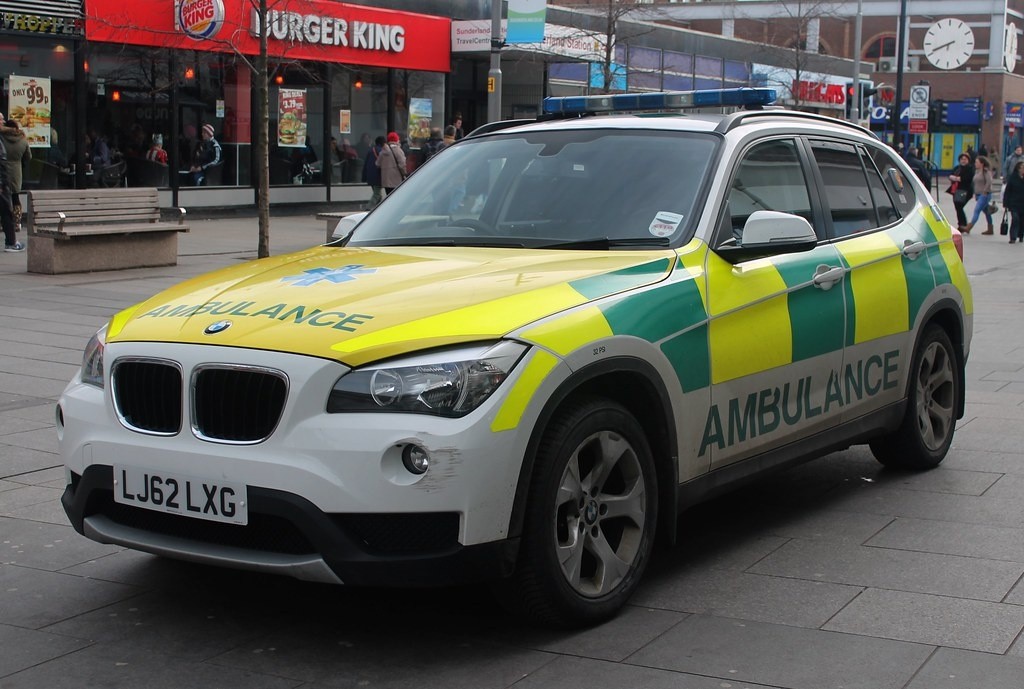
[
  {"xmin": 314, "ymin": 211, "xmax": 450, "ymax": 247},
  {"xmin": 25, "ymin": 190, "xmax": 189, "ymax": 276}
]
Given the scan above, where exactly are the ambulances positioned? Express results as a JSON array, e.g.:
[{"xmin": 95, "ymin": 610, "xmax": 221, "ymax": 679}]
[{"xmin": 52, "ymin": 85, "xmax": 977, "ymax": 647}]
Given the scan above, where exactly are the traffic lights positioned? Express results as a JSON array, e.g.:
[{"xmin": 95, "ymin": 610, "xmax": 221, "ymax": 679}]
[{"xmin": 847, "ymin": 85, "xmax": 878, "ymax": 118}]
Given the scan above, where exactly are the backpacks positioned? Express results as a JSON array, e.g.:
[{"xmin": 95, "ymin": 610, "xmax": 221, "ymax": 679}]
[{"xmin": 425, "ymin": 141, "xmax": 444, "ymax": 161}]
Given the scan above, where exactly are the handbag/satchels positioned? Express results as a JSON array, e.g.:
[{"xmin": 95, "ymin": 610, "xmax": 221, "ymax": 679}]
[
  {"xmin": 983, "ymin": 201, "xmax": 998, "ymax": 214},
  {"xmin": 953, "ymin": 189, "xmax": 967, "ymax": 203},
  {"xmin": 1001, "ymin": 210, "xmax": 1008, "ymax": 235},
  {"xmin": 402, "ymin": 176, "xmax": 406, "ymax": 181}
]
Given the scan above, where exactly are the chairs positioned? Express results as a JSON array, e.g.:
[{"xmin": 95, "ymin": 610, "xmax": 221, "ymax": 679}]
[
  {"xmin": 100, "ymin": 153, "xmax": 225, "ymax": 187},
  {"xmin": 322, "ymin": 158, "xmax": 366, "ymax": 183}
]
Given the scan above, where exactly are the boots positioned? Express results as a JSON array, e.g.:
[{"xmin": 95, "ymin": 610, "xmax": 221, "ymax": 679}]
[
  {"xmin": 982, "ymin": 225, "xmax": 993, "ymax": 234},
  {"xmin": 960, "ymin": 223, "xmax": 973, "ymax": 233}
]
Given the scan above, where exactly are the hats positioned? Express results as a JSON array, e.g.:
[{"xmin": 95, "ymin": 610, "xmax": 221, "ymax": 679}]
[
  {"xmin": 202, "ymin": 124, "xmax": 214, "ymax": 136},
  {"xmin": 376, "ymin": 137, "xmax": 386, "ymax": 143},
  {"xmin": 152, "ymin": 134, "xmax": 163, "ymax": 144},
  {"xmin": 388, "ymin": 132, "xmax": 398, "ymax": 141}
]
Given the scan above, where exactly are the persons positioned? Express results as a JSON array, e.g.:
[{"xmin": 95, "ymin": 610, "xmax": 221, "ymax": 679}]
[
  {"xmin": 899, "ymin": 145, "xmax": 1024, "ymax": 245},
  {"xmin": 0, "ymin": 113, "xmax": 463, "ymax": 252}
]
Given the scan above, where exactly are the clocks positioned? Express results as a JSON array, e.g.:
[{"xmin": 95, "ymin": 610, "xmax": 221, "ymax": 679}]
[
  {"xmin": 1004, "ymin": 22, "xmax": 1018, "ymax": 72},
  {"xmin": 923, "ymin": 18, "xmax": 975, "ymax": 70}
]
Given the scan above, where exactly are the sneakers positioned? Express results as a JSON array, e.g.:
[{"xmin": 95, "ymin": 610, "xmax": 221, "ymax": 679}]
[{"xmin": 4, "ymin": 240, "xmax": 25, "ymax": 252}]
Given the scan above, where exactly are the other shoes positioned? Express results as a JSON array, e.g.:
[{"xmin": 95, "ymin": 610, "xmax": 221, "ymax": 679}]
[
  {"xmin": 16, "ymin": 224, "xmax": 20, "ymax": 232},
  {"xmin": 1019, "ymin": 237, "xmax": 1023, "ymax": 242},
  {"xmin": 1009, "ymin": 239, "xmax": 1015, "ymax": 243}
]
